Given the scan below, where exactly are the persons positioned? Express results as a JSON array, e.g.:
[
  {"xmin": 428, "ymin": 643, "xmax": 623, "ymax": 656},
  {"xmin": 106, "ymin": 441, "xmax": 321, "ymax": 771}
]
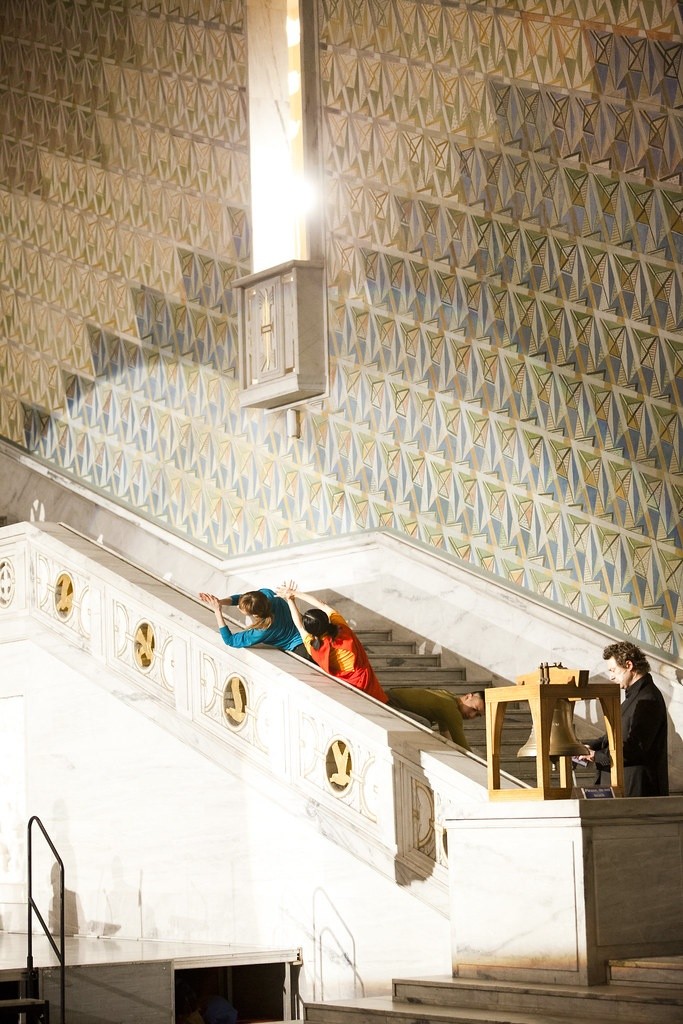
[
  {"xmin": 387, "ymin": 686, "xmax": 487, "ymax": 752},
  {"xmin": 276, "ymin": 581, "xmax": 389, "ymax": 707},
  {"xmin": 199, "ymin": 589, "xmax": 317, "ymax": 665},
  {"xmin": 571, "ymin": 642, "xmax": 669, "ymax": 795}
]
